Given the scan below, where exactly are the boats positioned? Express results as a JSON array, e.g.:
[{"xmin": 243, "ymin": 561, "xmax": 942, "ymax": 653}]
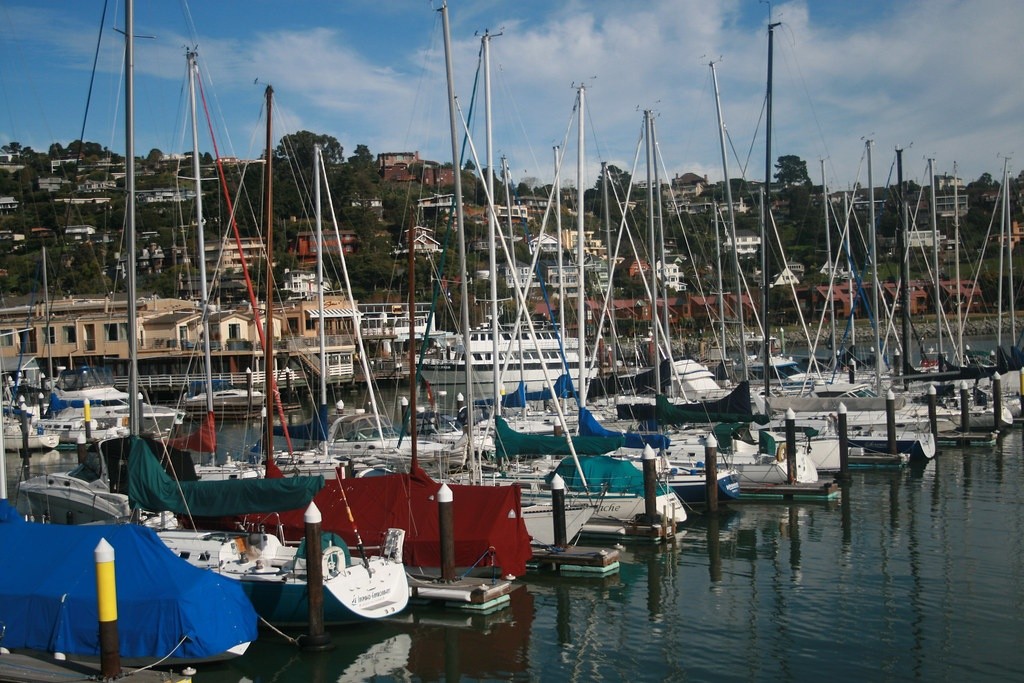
[
  {"xmin": 414, "ymin": 321, "xmax": 600, "ymax": 384},
  {"xmin": 0, "ymin": 0, "xmax": 1021, "ymax": 617},
  {"xmin": 49, "ymin": 384, "xmax": 145, "ymax": 406},
  {"xmin": 182, "ymin": 388, "xmax": 267, "ymax": 420}
]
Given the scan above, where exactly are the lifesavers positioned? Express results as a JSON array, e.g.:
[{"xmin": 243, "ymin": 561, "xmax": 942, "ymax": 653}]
[
  {"xmin": 321, "ymin": 546, "xmax": 346, "ymax": 578},
  {"xmin": 122, "ymin": 417, "xmax": 129, "ymax": 426},
  {"xmin": 776, "ymin": 443, "xmax": 788, "ymax": 462}
]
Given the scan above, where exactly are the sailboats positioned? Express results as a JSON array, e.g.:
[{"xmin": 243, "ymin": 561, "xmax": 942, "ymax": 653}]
[
  {"xmin": 2, "ymin": 83, "xmax": 260, "ymax": 670},
  {"xmin": 62, "ymin": 1, "xmax": 411, "ymax": 637}
]
